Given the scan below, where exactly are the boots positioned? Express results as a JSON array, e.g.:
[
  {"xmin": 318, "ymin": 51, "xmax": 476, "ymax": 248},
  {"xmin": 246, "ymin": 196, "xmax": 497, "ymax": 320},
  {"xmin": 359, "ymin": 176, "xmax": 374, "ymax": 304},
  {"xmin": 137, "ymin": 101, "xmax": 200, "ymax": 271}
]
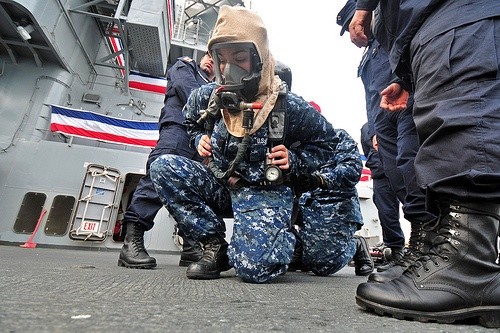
[
  {"xmin": 357, "ymin": 203, "xmax": 500, "ymax": 328},
  {"xmin": 177, "ymin": 230, "xmax": 204, "ymax": 266},
  {"xmin": 368, "ymin": 223, "xmax": 437, "ymax": 282},
  {"xmin": 286, "ymin": 223, "xmax": 306, "ymax": 273},
  {"xmin": 377, "ymin": 246, "xmax": 404, "ymax": 272},
  {"xmin": 186, "ymin": 236, "xmax": 234, "ymax": 280},
  {"xmin": 352, "ymin": 235, "xmax": 374, "ymax": 276},
  {"xmin": 118, "ymin": 220, "xmax": 157, "ymax": 269}
]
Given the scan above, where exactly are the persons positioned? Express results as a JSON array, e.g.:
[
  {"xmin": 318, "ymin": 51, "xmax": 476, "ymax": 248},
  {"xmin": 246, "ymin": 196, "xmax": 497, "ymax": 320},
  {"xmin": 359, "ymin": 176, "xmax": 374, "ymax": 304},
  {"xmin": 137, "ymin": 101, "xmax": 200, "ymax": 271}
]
[
  {"xmin": 118, "ymin": 50, "xmax": 216, "ymax": 269},
  {"xmin": 361, "ymin": 122, "xmax": 403, "ymax": 272},
  {"xmin": 336, "ymin": 0, "xmax": 439, "ymax": 283},
  {"xmin": 349, "ymin": 0, "xmax": 500, "ymax": 329},
  {"xmin": 149, "ymin": 7, "xmax": 340, "ymax": 283},
  {"xmin": 271, "ymin": 59, "xmax": 374, "ymax": 277}
]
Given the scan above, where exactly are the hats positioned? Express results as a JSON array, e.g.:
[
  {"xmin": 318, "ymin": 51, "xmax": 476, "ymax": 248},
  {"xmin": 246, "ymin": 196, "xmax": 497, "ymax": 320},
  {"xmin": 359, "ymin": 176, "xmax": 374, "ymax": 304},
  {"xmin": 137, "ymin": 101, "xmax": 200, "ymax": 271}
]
[{"xmin": 336, "ymin": 0, "xmax": 357, "ymax": 36}]
